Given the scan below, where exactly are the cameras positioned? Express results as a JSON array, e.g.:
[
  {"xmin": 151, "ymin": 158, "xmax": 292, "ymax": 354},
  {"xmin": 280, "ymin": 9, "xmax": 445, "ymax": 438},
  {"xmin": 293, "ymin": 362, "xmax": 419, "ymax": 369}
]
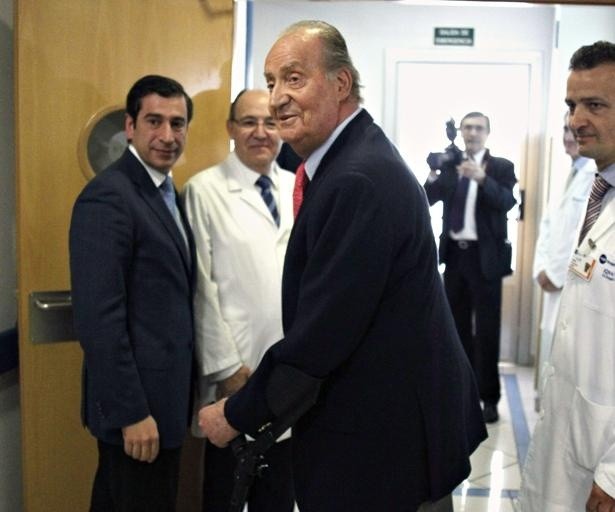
[{"xmin": 426, "ymin": 115, "xmax": 463, "ymax": 170}]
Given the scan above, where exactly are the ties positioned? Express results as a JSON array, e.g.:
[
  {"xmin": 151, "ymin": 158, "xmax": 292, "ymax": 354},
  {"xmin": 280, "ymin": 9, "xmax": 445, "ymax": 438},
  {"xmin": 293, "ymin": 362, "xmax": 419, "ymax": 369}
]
[
  {"xmin": 450, "ymin": 175, "xmax": 470, "ymax": 233},
  {"xmin": 160, "ymin": 176, "xmax": 177, "ymax": 224},
  {"xmin": 293, "ymin": 161, "xmax": 306, "ymax": 222},
  {"xmin": 255, "ymin": 175, "xmax": 279, "ymax": 227},
  {"xmin": 577, "ymin": 175, "xmax": 613, "ymax": 247}
]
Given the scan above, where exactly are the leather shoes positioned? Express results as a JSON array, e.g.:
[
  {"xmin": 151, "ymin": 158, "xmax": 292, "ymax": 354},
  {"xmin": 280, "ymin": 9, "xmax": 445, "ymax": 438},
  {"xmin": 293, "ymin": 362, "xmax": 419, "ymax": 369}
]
[{"xmin": 482, "ymin": 400, "xmax": 499, "ymax": 424}]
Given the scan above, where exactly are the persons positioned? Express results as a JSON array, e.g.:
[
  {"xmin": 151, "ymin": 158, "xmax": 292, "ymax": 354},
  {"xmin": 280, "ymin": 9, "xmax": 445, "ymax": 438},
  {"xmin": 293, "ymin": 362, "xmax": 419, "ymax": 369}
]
[
  {"xmin": 193, "ymin": 16, "xmax": 490, "ymax": 512},
  {"xmin": 68, "ymin": 72, "xmax": 197, "ymax": 512},
  {"xmin": 531, "ymin": 107, "xmax": 599, "ymax": 418},
  {"xmin": 510, "ymin": 37, "xmax": 615, "ymax": 512},
  {"xmin": 182, "ymin": 85, "xmax": 301, "ymax": 512},
  {"xmin": 423, "ymin": 108, "xmax": 517, "ymax": 425}
]
